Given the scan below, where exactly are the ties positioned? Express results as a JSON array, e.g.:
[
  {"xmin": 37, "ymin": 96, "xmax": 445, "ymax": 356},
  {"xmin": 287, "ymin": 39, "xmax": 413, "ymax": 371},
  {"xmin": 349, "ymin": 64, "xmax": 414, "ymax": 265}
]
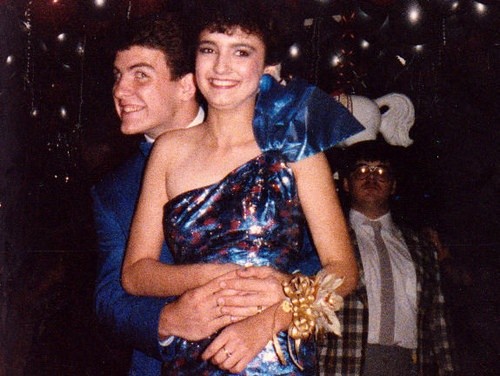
[{"xmin": 362, "ymin": 220, "xmax": 395, "ymax": 346}]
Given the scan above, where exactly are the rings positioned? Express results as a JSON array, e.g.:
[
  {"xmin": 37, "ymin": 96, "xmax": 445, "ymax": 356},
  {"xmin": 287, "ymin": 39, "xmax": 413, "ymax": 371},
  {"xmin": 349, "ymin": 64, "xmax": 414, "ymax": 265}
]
[
  {"xmin": 222, "ymin": 347, "xmax": 233, "ymax": 357},
  {"xmin": 257, "ymin": 306, "xmax": 262, "ymax": 314}
]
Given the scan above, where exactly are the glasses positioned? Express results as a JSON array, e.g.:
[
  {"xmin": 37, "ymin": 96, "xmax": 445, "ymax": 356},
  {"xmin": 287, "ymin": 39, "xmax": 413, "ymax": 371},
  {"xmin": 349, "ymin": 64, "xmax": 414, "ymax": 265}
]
[{"xmin": 347, "ymin": 164, "xmax": 396, "ymax": 182}]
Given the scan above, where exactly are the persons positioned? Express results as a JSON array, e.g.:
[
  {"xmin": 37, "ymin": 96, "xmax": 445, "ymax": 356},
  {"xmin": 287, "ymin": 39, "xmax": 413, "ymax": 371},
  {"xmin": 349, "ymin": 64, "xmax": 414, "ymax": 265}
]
[
  {"xmin": 419, "ymin": 217, "xmax": 468, "ymax": 365},
  {"xmin": 301, "ymin": 140, "xmax": 461, "ymax": 376},
  {"xmin": 88, "ymin": 7, "xmax": 362, "ymax": 375}
]
